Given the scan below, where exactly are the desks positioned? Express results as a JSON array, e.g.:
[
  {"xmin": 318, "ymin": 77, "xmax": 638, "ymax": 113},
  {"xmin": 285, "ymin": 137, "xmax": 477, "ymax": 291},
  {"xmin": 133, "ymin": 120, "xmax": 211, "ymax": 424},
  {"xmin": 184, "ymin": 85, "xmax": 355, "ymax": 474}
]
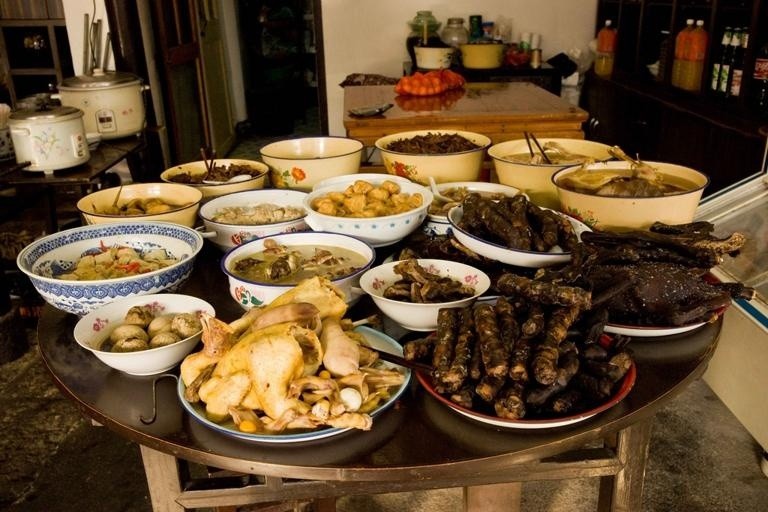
[
  {"xmin": 344, "ymin": 81, "xmax": 590, "ymax": 166},
  {"xmin": 36, "ymin": 240, "xmax": 724, "ymax": 512},
  {"xmin": 0, "ymin": 136, "xmax": 145, "ymax": 233}
]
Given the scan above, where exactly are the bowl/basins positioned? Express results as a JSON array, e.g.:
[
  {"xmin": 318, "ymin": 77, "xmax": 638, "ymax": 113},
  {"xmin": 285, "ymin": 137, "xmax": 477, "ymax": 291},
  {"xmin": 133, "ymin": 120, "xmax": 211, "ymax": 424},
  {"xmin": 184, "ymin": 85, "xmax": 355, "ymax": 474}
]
[
  {"xmin": 259, "ymin": 138, "xmax": 362, "ymax": 189},
  {"xmin": 488, "ymin": 138, "xmax": 709, "ymax": 233},
  {"xmin": 74, "ymin": 184, "xmax": 203, "ymax": 228},
  {"xmin": 221, "ymin": 231, "xmax": 373, "ymax": 314},
  {"xmin": 160, "ymin": 159, "xmax": 272, "ymax": 199},
  {"xmin": 199, "ymin": 189, "xmax": 310, "ymax": 247},
  {"xmin": 414, "ymin": 43, "xmax": 503, "ymax": 72},
  {"xmin": 302, "ymin": 173, "xmax": 435, "ymax": 248},
  {"xmin": 361, "ymin": 259, "xmax": 491, "ymax": 331},
  {"xmin": 18, "ymin": 223, "xmax": 203, "ymax": 314},
  {"xmin": 70, "ymin": 294, "xmax": 216, "ymax": 375},
  {"xmin": 375, "ymin": 129, "xmax": 492, "ymax": 186}
]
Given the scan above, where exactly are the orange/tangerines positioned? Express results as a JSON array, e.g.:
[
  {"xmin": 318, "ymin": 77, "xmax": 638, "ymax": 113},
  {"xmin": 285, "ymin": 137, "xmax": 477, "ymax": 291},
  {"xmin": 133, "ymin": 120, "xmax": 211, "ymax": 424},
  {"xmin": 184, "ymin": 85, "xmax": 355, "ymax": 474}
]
[
  {"xmin": 394, "ymin": 71, "xmax": 448, "ymax": 96},
  {"xmin": 394, "ymin": 91, "xmax": 452, "ymax": 111}
]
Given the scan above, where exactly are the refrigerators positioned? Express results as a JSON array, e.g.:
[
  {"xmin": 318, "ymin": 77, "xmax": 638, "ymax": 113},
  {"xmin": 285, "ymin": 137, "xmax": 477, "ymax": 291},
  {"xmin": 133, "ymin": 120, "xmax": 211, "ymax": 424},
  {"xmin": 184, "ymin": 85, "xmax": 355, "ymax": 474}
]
[{"xmin": 690, "ymin": 144, "xmax": 768, "ymax": 467}]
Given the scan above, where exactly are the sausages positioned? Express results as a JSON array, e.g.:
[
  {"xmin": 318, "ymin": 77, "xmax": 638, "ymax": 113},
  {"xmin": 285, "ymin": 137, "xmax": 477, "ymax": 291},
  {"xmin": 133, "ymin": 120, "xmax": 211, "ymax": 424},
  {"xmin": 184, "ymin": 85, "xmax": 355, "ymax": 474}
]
[
  {"xmin": 456, "ymin": 193, "xmax": 579, "ymax": 256},
  {"xmin": 403, "ymin": 274, "xmax": 632, "ymax": 420}
]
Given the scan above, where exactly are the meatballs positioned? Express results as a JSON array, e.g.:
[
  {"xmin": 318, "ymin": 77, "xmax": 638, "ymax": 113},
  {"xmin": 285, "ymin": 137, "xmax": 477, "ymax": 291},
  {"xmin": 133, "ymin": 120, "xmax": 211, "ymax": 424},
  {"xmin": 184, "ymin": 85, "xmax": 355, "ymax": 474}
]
[{"xmin": 110, "ymin": 304, "xmax": 203, "ymax": 354}]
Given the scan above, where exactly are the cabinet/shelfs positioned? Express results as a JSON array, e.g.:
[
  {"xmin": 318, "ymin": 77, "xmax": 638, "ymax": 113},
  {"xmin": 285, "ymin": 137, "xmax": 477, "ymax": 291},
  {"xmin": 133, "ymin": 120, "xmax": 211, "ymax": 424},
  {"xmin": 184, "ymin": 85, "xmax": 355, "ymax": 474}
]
[{"xmin": 581, "ymin": 0, "xmax": 768, "ymax": 194}]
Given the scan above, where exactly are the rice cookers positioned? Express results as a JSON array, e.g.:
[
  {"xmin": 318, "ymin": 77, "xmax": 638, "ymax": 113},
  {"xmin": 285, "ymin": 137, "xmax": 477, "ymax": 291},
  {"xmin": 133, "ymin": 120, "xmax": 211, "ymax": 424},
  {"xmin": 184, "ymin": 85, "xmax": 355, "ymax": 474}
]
[{"xmin": 6, "ymin": 67, "xmax": 151, "ymax": 177}]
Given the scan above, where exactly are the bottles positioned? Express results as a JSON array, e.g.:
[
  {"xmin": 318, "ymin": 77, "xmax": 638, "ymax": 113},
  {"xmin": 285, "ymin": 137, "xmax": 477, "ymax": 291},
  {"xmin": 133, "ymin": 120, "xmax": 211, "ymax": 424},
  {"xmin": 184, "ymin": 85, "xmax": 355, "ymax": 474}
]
[
  {"xmin": 406, "ymin": 10, "xmax": 493, "ymax": 43},
  {"xmin": 595, "ymin": 20, "xmax": 617, "ymax": 79},
  {"xmin": 659, "ymin": 17, "xmax": 768, "ymax": 106}
]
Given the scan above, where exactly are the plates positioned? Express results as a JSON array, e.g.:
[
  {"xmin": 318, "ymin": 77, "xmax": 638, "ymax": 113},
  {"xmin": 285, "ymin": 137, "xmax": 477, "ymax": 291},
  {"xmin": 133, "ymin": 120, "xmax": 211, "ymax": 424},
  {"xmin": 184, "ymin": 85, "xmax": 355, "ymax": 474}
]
[
  {"xmin": 428, "ymin": 183, "xmax": 520, "ymax": 230},
  {"xmin": 448, "ymin": 205, "xmax": 596, "ymax": 267},
  {"xmin": 603, "ymin": 265, "xmax": 731, "ymax": 336},
  {"xmin": 413, "ymin": 331, "xmax": 639, "ymax": 428},
  {"xmin": 177, "ymin": 322, "xmax": 411, "ymax": 445}
]
